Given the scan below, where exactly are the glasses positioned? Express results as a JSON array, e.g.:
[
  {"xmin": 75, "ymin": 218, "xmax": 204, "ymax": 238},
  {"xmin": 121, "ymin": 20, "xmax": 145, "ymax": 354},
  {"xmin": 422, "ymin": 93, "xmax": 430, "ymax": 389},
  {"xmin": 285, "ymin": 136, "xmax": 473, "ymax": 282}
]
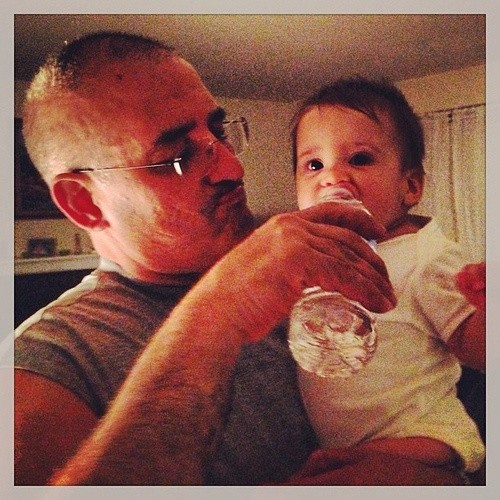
[{"xmin": 70, "ymin": 114, "xmax": 250, "ymax": 183}]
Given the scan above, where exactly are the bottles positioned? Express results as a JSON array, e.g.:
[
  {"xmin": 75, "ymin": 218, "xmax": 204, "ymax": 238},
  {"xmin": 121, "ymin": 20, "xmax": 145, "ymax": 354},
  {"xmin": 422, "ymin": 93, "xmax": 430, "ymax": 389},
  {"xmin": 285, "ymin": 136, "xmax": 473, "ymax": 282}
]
[{"xmin": 287, "ymin": 195, "xmax": 379, "ymax": 381}]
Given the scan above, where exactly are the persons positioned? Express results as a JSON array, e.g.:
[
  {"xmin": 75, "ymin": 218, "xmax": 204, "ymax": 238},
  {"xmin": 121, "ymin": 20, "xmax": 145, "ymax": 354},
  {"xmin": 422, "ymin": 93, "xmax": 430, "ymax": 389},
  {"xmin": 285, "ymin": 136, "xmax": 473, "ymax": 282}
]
[
  {"xmin": 285, "ymin": 74, "xmax": 485, "ymax": 472},
  {"xmin": 14, "ymin": 29, "xmax": 467, "ymax": 485}
]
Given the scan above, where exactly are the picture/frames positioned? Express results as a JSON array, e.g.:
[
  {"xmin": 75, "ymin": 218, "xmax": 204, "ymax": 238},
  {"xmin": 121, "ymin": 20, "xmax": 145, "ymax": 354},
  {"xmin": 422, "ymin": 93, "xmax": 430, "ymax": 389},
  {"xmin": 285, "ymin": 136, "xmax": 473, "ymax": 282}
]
[{"xmin": 13, "ymin": 115, "xmax": 70, "ymax": 220}]
